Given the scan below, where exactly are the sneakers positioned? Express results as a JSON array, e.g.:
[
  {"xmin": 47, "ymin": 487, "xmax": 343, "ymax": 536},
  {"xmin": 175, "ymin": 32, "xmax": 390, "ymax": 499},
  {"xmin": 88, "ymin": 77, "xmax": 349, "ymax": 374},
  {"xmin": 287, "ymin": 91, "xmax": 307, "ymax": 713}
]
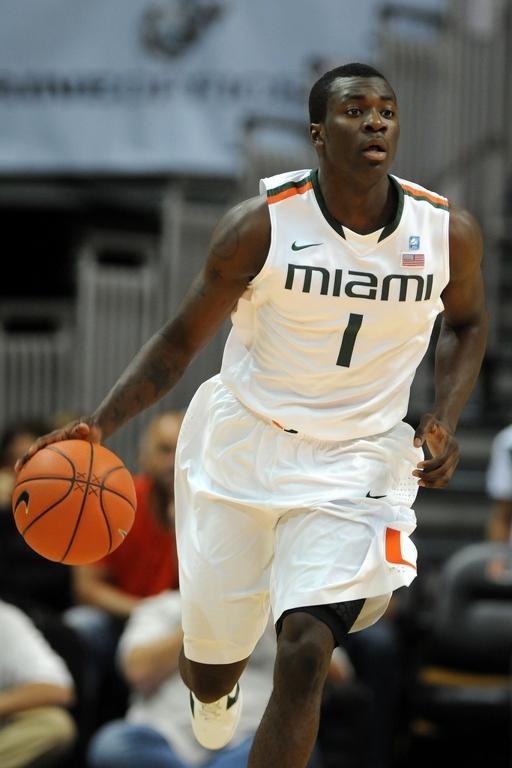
[{"xmin": 186, "ymin": 683, "xmax": 241, "ymax": 751}]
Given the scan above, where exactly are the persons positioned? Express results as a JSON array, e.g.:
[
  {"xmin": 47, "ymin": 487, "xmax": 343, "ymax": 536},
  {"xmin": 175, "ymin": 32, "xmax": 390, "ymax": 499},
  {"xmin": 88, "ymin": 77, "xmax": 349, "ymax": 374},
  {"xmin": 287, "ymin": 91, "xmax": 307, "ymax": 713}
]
[
  {"xmin": 12, "ymin": 60, "xmax": 491, "ymax": 768},
  {"xmin": 1, "ymin": 407, "xmax": 512, "ymax": 768}
]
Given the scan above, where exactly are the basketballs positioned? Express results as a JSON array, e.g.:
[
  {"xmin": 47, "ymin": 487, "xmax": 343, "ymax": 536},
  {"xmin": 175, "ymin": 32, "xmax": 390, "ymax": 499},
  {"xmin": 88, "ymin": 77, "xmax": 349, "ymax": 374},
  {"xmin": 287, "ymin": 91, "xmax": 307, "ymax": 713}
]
[{"xmin": 13, "ymin": 440, "xmax": 139, "ymax": 565}]
